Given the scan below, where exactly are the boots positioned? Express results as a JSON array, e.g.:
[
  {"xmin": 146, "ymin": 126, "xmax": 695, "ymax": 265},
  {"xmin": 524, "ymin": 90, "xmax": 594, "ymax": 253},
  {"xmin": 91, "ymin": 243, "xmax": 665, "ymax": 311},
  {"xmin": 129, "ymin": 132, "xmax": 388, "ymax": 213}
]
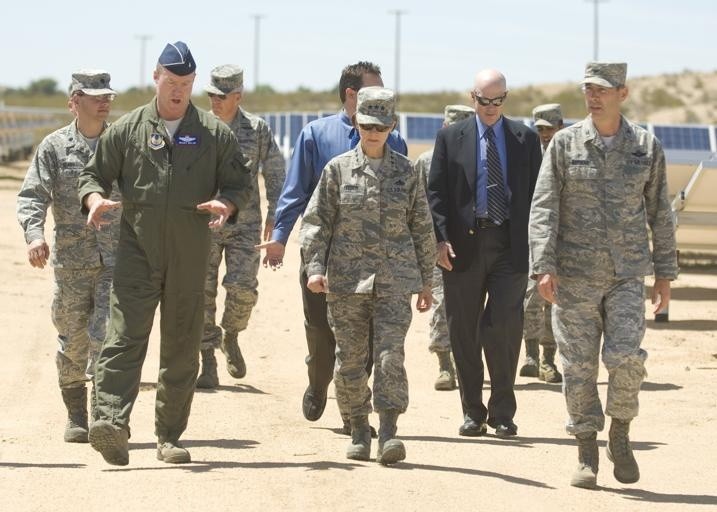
[
  {"xmin": 218, "ymin": 329, "xmax": 246, "ymax": 378},
  {"xmin": 435, "ymin": 349, "xmax": 456, "ymax": 390},
  {"xmin": 87, "ymin": 419, "xmax": 129, "ymax": 466},
  {"xmin": 377, "ymin": 409, "xmax": 407, "ymax": 466},
  {"xmin": 157, "ymin": 434, "xmax": 190, "ymax": 463},
  {"xmin": 606, "ymin": 418, "xmax": 640, "ymax": 483},
  {"xmin": 520, "ymin": 338, "xmax": 539, "ymax": 377},
  {"xmin": 571, "ymin": 431, "xmax": 599, "ymax": 488},
  {"xmin": 347, "ymin": 416, "xmax": 372, "ymax": 462},
  {"xmin": 539, "ymin": 345, "xmax": 562, "ymax": 382},
  {"xmin": 62, "ymin": 387, "xmax": 89, "ymax": 443},
  {"xmin": 196, "ymin": 348, "xmax": 219, "ymax": 388}
]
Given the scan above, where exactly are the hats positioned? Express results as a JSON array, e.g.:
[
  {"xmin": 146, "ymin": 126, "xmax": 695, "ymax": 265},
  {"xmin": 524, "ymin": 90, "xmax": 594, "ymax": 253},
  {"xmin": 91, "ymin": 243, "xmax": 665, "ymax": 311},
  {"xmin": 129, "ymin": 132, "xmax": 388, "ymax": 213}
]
[
  {"xmin": 159, "ymin": 42, "xmax": 196, "ymax": 76},
  {"xmin": 532, "ymin": 103, "xmax": 562, "ymax": 127},
  {"xmin": 71, "ymin": 72, "xmax": 118, "ymax": 97},
  {"xmin": 207, "ymin": 65, "xmax": 243, "ymax": 96},
  {"xmin": 445, "ymin": 105, "xmax": 475, "ymax": 127},
  {"xmin": 356, "ymin": 85, "xmax": 397, "ymax": 127},
  {"xmin": 581, "ymin": 63, "xmax": 627, "ymax": 88}
]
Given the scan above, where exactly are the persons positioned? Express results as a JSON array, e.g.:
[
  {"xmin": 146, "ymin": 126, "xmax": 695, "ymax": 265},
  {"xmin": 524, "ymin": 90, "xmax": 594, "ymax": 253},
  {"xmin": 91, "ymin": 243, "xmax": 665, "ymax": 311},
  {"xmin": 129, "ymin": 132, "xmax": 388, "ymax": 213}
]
[
  {"xmin": 417, "ymin": 105, "xmax": 478, "ymax": 390},
  {"xmin": 66, "ymin": 84, "xmax": 76, "ymax": 120},
  {"xmin": 530, "ymin": 59, "xmax": 680, "ymax": 487},
  {"xmin": 296, "ymin": 86, "xmax": 438, "ymax": 466},
  {"xmin": 430, "ymin": 71, "xmax": 544, "ymax": 435},
  {"xmin": 198, "ymin": 66, "xmax": 288, "ymax": 389},
  {"xmin": 19, "ymin": 70, "xmax": 133, "ymax": 443},
  {"xmin": 519, "ymin": 101, "xmax": 565, "ymax": 383},
  {"xmin": 253, "ymin": 60, "xmax": 410, "ymax": 437},
  {"xmin": 80, "ymin": 42, "xmax": 254, "ymax": 467}
]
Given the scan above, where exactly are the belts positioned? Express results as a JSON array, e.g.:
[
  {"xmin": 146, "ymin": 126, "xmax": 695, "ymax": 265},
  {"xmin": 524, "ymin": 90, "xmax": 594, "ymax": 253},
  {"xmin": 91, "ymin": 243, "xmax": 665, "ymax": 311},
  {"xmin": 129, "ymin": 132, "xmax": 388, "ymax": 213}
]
[{"xmin": 473, "ymin": 218, "xmax": 510, "ymax": 229}]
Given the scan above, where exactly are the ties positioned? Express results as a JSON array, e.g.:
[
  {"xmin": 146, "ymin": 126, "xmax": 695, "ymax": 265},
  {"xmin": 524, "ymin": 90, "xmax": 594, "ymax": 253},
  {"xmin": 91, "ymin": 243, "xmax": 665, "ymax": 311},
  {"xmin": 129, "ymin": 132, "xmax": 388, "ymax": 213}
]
[
  {"xmin": 350, "ymin": 128, "xmax": 360, "ymax": 149},
  {"xmin": 484, "ymin": 127, "xmax": 507, "ymax": 226}
]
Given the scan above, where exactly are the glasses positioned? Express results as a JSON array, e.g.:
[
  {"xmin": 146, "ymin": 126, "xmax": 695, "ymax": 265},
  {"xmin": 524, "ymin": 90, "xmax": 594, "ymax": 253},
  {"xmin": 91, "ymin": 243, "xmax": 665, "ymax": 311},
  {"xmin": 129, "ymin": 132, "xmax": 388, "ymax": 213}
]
[
  {"xmin": 207, "ymin": 93, "xmax": 227, "ymax": 100},
  {"xmin": 85, "ymin": 94, "xmax": 116, "ymax": 101},
  {"xmin": 475, "ymin": 92, "xmax": 506, "ymax": 106},
  {"xmin": 358, "ymin": 124, "xmax": 391, "ymax": 132}
]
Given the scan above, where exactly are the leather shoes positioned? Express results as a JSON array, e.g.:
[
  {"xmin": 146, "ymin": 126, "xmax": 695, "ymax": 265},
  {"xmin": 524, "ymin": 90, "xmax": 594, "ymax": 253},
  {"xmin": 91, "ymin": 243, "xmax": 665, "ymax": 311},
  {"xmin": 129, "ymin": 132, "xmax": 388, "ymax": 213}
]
[
  {"xmin": 496, "ymin": 423, "xmax": 518, "ymax": 436},
  {"xmin": 459, "ymin": 420, "xmax": 487, "ymax": 436},
  {"xmin": 302, "ymin": 383, "xmax": 327, "ymax": 421}
]
